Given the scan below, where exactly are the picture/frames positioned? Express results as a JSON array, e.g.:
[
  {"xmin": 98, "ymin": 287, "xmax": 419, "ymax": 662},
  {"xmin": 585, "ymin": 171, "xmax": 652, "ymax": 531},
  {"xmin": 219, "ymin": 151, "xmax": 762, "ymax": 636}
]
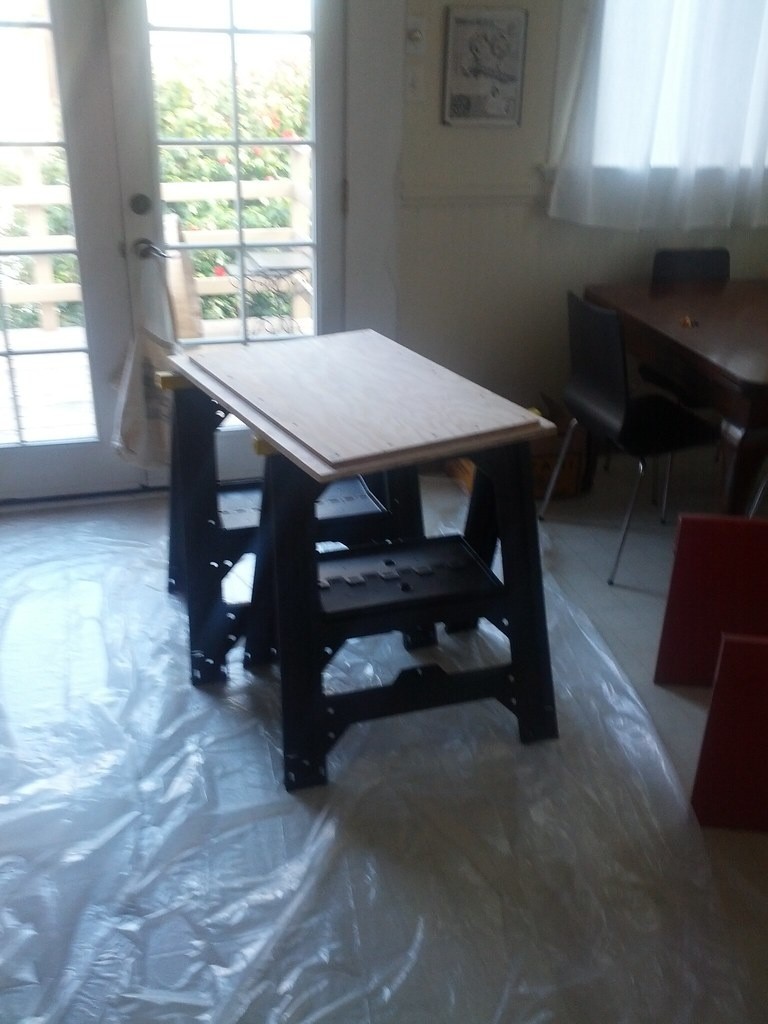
[{"xmin": 442, "ymin": 6, "xmax": 528, "ymax": 126}]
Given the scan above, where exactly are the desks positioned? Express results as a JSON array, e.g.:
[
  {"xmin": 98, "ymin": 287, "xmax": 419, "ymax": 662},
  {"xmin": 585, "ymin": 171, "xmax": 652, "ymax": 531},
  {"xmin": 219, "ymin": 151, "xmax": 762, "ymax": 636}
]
[
  {"xmin": 585, "ymin": 282, "xmax": 768, "ymax": 514},
  {"xmin": 157, "ymin": 329, "xmax": 556, "ymax": 791}
]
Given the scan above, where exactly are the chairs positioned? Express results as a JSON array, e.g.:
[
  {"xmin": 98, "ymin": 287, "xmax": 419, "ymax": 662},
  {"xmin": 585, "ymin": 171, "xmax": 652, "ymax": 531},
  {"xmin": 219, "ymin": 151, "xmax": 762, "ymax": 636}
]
[
  {"xmin": 538, "ymin": 289, "xmax": 718, "ymax": 584},
  {"xmin": 639, "ymin": 245, "xmax": 730, "ymax": 505}
]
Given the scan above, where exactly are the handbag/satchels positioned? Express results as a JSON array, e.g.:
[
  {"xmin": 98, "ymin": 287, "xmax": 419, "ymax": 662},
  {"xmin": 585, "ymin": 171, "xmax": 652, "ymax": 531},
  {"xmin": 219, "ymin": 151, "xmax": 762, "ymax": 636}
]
[{"xmin": 112, "ymin": 240, "xmax": 182, "ymax": 471}]
